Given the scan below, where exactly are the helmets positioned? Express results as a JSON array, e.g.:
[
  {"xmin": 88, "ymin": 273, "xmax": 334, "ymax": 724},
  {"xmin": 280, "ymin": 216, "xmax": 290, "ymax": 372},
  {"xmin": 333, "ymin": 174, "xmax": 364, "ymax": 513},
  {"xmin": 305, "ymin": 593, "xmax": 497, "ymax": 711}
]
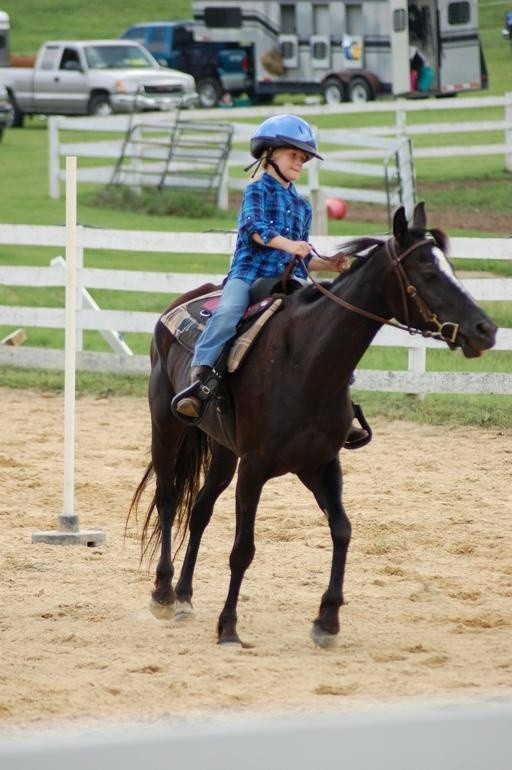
[{"xmin": 251, "ymin": 114, "xmax": 324, "ymax": 163}]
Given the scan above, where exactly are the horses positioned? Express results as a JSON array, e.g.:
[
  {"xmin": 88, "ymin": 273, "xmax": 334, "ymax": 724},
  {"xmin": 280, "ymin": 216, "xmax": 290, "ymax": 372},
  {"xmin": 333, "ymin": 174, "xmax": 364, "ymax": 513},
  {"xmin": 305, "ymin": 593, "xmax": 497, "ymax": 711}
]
[{"xmin": 119, "ymin": 199, "xmax": 500, "ymax": 649}]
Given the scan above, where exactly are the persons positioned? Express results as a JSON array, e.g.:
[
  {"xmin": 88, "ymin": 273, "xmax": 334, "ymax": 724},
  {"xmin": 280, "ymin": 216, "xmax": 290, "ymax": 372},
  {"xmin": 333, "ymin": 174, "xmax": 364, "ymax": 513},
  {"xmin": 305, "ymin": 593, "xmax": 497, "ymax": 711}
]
[{"xmin": 175, "ymin": 115, "xmax": 370, "ymax": 446}]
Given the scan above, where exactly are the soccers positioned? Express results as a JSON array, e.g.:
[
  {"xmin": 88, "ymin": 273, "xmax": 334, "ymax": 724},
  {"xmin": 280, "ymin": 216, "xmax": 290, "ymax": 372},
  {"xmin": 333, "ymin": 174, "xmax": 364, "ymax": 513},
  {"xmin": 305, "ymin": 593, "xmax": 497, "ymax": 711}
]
[{"xmin": 326, "ymin": 199, "xmax": 346, "ymax": 218}]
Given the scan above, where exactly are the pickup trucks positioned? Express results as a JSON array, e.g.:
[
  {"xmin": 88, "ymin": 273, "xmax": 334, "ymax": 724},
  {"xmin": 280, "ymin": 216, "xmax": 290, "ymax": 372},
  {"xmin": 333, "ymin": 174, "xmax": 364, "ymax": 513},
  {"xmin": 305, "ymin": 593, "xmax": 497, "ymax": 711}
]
[
  {"xmin": 119, "ymin": 23, "xmax": 273, "ymax": 109},
  {"xmin": 1, "ymin": 39, "xmax": 199, "ymax": 121}
]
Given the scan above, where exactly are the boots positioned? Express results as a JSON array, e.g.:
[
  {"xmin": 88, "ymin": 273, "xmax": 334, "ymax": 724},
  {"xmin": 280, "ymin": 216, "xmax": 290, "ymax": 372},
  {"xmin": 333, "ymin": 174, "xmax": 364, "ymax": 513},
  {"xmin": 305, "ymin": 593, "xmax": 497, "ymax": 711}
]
[
  {"xmin": 346, "ymin": 424, "xmax": 369, "ymax": 444},
  {"xmin": 176, "ymin": 367, "xmax": 209, "ymax": 417}
]
